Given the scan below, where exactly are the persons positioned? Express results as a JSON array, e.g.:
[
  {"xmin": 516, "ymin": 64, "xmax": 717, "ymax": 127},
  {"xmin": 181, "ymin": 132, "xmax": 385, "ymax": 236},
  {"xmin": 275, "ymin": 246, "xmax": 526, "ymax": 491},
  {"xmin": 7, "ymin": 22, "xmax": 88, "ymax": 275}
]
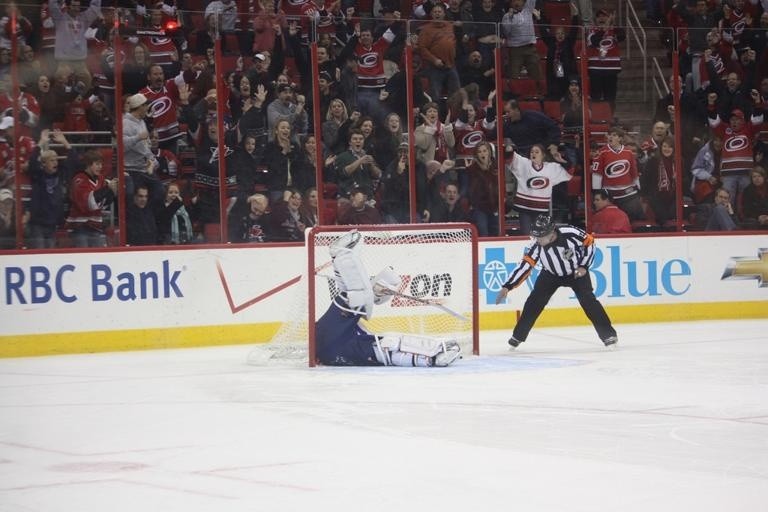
[
  {"xmin": 313, "ymin": 229, "xmax": 460, "ymax": 367},
  {"xmin": 496, "ymin": 214, "xmax": 618, "ymax": 348}
]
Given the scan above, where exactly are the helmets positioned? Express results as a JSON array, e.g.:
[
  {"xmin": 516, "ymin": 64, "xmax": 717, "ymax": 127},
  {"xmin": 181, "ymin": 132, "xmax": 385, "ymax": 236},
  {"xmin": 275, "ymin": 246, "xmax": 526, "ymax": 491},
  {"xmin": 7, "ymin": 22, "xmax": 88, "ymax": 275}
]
[{"xmin": 526, "ymin": 213, "xmax": 556, "ymax": 237}]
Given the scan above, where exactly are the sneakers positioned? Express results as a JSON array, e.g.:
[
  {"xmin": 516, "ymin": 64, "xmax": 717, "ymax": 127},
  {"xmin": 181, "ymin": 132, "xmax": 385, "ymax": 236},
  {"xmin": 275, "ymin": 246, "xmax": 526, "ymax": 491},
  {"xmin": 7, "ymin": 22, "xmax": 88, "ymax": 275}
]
[
  {"xmin": 433, "ymin": 343, "xmax": 461, "ymax": 366},
  {"xmin": 603, "ymin": 336, "xmax": 619, "ymax": 345},
  {"xmin": 508, "ymin": 336, "xmax": 522, "ymax": 347},
  {"xmin": 329, "ymin": 231, "xmax": 362, "ymax": 259}
]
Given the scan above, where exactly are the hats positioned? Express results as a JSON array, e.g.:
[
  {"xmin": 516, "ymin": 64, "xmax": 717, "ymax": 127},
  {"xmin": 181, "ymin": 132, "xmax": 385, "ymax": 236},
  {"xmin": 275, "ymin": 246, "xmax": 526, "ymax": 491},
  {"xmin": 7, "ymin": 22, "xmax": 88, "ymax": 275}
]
[
  {"xmin": 252, "ymin": 53, "xmax": 265, "ymax": 62},
  {"xmin": 129, "ymin": 93, "xmax": 148, "ymax": 110},
  {"xmin": 0, "ymin": 116, "xmax": 15, "ymax": 130},
  {"xmin": 729, "ymin": 108, "xmax": 745, "ymax": 120},
  {"xmin": 0, "ymin": 189, "xmax": 13, "ymax": 202}
]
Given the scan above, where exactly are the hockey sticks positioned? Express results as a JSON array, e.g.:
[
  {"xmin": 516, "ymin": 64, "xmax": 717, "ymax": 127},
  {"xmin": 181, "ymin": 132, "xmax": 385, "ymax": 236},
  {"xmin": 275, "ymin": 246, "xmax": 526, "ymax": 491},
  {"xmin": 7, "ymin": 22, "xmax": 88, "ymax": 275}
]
[{"xmin": 313, "ymin": 271, "xmax": 472, "ymax": 323}]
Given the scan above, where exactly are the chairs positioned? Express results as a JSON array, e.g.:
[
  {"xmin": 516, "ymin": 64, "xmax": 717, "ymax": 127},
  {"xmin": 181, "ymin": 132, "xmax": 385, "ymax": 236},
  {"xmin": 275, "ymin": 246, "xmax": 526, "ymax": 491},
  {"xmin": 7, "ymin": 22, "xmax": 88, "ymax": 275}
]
[{"xmin": 0, "ymin": 1, "xmax": 767, "ymax": 248}]
